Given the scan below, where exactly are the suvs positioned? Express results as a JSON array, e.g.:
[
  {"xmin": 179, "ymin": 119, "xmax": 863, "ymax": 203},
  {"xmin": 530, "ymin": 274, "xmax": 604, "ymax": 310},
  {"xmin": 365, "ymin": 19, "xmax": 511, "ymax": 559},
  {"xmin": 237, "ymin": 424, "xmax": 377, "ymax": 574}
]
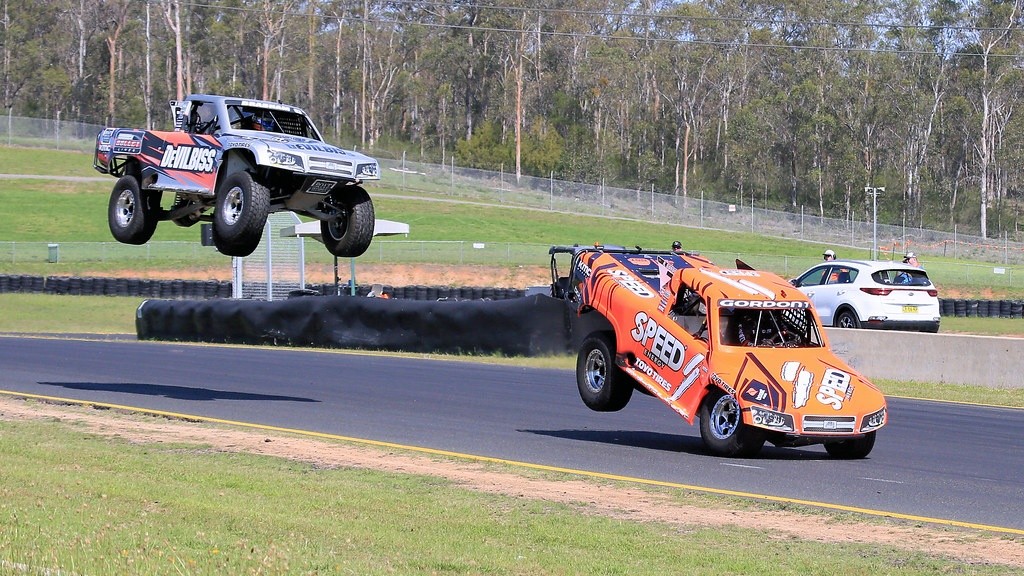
[
  {"xmin": 547, "ymin": 241, "xmax": 888, "ymax": 461},
  {"xmin": 786, "ymin": 260, "xmax": 940, "ymax": 333},
  {"xmin": 94, "ymin": 94, "xmax": 381, "ymax": 258}
]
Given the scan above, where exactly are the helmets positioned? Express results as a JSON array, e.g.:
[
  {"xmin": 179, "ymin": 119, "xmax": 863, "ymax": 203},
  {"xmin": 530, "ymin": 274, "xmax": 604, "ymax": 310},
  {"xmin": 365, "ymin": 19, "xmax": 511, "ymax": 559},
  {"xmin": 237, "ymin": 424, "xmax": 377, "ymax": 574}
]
[
  {"xmin": 822, "ymin": 250, "xmax": 834, "ymax": 259},
  {"xmin": 252, "ymin": 112, "xmax": 275, "ymax": 131}
]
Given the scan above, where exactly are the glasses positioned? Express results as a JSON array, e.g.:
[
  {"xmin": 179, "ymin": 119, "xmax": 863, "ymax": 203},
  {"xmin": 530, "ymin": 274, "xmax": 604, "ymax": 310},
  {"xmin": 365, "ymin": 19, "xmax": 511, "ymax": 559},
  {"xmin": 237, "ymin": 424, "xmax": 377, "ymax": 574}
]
[{"xmin": 907, "ymin": 258, "xmax": 910, "ymax": 260}]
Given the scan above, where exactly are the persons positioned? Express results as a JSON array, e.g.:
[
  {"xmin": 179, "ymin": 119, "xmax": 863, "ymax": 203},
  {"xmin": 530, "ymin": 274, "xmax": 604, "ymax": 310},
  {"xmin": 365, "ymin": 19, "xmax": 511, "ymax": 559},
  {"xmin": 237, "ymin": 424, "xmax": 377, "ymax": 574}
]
[
  {"xmin": 821, "ymin": 250, "xmax": 850, "ymax": 284},
  {"xmin": 736, "ymin": 308, "xmax": 796, "ymax": 347},
  {"xmin": 250, "ymin": 109, "xmax": 276, "ymax": 132},
  {"xmin": 894, "ymin": 253, "xmax": 928, "ymax": 285},
  {"xmin": 673, "ymin": 240, "xmax": 682, "ymax": 251}
]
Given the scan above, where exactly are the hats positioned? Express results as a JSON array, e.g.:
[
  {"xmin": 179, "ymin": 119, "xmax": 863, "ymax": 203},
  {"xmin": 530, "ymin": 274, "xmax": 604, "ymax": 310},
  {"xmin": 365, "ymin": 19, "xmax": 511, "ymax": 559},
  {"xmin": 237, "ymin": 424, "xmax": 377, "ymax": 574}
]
[
  {"xmin": 672, "ymin": 241, "xmax": 682, "ymax": 248},
  {"xmin": 903, "ymin": 252, "xmax": 916, "ymax": 258}
]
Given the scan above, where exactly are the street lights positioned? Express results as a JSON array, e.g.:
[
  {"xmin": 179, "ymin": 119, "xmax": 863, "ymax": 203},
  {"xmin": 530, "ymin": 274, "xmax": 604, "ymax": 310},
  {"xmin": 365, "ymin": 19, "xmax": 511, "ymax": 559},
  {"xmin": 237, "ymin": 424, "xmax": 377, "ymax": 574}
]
[{"xmin": 864, "ymin": 186, "xmax": 885, "ymax": 261}]
